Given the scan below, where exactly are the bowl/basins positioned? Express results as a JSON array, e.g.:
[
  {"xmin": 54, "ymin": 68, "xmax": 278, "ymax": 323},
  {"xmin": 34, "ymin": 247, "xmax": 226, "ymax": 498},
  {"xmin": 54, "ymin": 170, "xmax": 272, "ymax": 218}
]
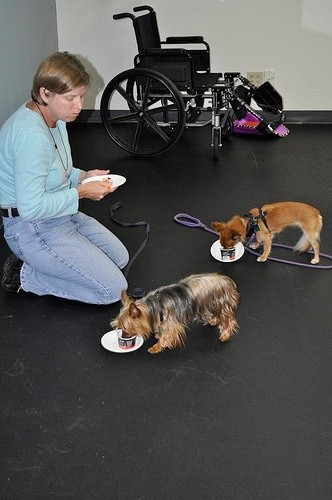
[
  {"xmin": 220, "ymin": 247, "xmax": 235, "ymax": 260},
  {"xmin": 117, "ymin": 334, "xmax": 137, "ymax": 349}
]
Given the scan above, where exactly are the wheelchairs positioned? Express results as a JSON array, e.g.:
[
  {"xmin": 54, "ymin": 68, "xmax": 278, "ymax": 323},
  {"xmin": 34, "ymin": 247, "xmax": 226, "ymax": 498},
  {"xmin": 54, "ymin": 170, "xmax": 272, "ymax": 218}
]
[{"xmin": 97, "ymin": 4, "xmax": 287, "ymax": 167}]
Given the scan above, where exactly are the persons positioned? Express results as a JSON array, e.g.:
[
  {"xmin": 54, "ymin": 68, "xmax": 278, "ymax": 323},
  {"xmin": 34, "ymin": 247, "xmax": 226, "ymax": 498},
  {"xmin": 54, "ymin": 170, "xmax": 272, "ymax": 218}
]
[{"xmin": 0, "ymin": 52, "xmax": 130, "ymax": 304}]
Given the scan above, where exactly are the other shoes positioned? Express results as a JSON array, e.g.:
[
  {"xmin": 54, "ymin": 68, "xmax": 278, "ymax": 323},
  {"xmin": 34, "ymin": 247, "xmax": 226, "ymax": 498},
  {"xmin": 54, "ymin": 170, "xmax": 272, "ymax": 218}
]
[{"xmin": 0, "ymin": 253, "xmax": 24, "ymax": 293}]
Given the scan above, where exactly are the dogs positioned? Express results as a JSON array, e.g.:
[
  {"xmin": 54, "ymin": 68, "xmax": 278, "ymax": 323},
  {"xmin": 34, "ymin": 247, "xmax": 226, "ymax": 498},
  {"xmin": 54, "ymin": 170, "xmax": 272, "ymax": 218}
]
[
  {"xmin": 211, "ymin": 201, "xmax": 323, "ymax": 264},
  {"xmin": 110, "ymin": 273, "xmax": 241, "ymax": 353}
]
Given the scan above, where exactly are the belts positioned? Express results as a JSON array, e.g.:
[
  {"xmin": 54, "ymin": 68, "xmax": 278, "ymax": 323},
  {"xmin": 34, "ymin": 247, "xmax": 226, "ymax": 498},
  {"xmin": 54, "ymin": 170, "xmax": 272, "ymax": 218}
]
[{"xmin": 0, "ymin": 207, "xmax": 20, "ymax": 219}]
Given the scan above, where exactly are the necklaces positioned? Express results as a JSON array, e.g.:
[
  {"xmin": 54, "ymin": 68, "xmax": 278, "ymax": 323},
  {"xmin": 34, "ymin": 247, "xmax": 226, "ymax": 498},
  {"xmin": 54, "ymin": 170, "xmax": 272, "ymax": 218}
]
[{"xmin": 34, "ymin": 101, "xmax": 69, "ymax": 178}]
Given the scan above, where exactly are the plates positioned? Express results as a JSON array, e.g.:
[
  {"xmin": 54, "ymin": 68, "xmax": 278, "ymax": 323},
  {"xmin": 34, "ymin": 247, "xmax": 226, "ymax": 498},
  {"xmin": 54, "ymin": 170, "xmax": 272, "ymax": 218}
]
[
  {"xmin": 210, "ymin": 238, "xmax": 245, "ymax": 262},
  {"xmin": 100, "ymin": 328, "xmax": 144, "ymax": 353},
  {"xmin": 81, "ymin": 174, "xmax": 126, "ymax": 188}
]
